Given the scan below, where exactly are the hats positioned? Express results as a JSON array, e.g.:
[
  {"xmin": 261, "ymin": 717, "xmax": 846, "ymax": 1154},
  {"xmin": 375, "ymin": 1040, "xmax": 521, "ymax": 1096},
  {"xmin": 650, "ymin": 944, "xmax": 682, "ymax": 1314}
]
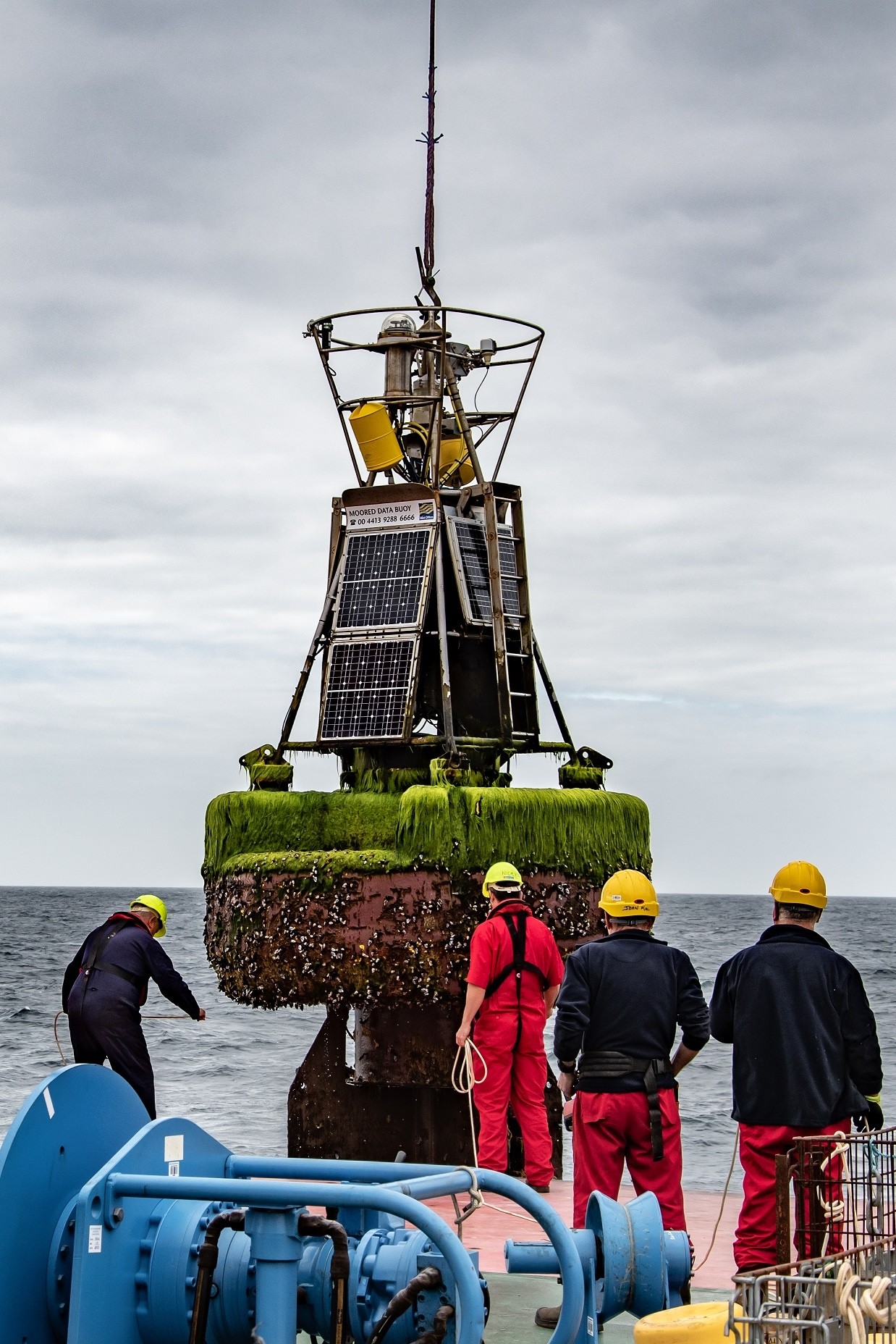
[{"xmin": 853, "ymin": 1094, "xmax": 885, "ymax": 1135}]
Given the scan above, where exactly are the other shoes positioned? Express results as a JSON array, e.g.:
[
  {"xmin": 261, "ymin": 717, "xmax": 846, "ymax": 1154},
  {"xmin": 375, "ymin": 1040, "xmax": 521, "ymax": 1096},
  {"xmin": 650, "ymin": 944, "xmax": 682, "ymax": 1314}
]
[
  {"xmin": 535, "ymin": 1304, "xmax": 604, "ymax": 1332},
  {"xmin": 525, "ymin": 1180, "xmax": 550, "ymax": 1193}
]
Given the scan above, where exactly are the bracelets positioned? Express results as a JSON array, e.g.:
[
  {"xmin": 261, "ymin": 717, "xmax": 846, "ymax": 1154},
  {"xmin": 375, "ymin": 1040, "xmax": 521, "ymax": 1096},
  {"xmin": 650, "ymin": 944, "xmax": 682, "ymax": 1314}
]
[
  {"xmin": 557, "ymin": 1059, "xmax": 576, "ymax": 1073},
  {"xmin": 864, "ymin": 1095, "xmax": 881, "ymax": 1103}
]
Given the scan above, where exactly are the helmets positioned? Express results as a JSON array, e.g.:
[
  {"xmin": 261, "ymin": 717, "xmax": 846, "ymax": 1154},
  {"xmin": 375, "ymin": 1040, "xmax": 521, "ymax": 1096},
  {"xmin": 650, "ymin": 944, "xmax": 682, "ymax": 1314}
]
[
  {"xmin": 482, "ymin": 861, "xmax": 524, "ymax": 898},
  {"xmin": 130, "ymin": 894, "xmax": 167, "ymax": 938},
  {"xmin": 598, "ymin": 869, "xmax": 659, "ymax": 917},
  {"xmin": 768, "ymin": 861, "xmax": 827, "ymax": 908}
]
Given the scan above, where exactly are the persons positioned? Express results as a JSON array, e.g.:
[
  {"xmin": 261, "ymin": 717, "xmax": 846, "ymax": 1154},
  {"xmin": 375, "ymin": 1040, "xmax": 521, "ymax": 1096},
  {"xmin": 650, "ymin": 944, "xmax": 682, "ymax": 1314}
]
[
  {"xmin": 535, "ymin": 868, "xmax": 711, "ymax": 1332},
  {"xmin": 454, "ymin": 862, "xmax": 565, "ymax": 1194},
  {"xmin": 710, "ymin": 859, "xmax": 884, "ymax": 1322},
  {"xmin": 62, "ymin": 894, "xmax": 206, "ymax": 1120}
]
